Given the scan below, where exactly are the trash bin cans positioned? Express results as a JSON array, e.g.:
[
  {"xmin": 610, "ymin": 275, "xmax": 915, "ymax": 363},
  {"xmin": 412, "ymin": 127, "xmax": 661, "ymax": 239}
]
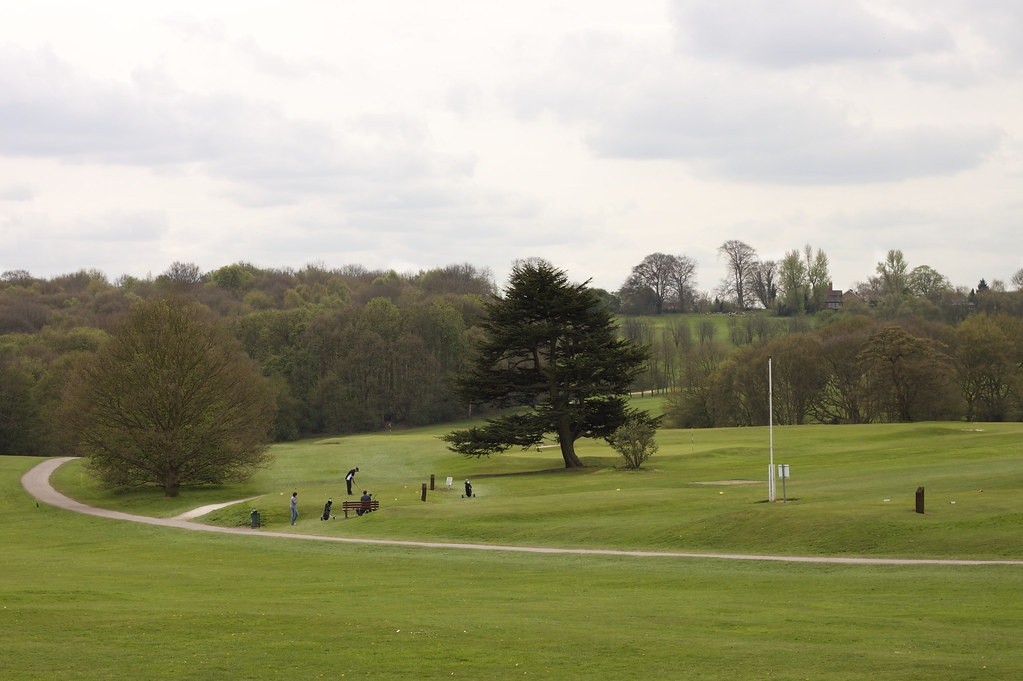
[
  {"xmin": 250, "ymin": 510, "xmax": 260, "ymax": 529},
  {"xmin": 915, "ymin": 487, "xmax": 925, "ymax": 513}
]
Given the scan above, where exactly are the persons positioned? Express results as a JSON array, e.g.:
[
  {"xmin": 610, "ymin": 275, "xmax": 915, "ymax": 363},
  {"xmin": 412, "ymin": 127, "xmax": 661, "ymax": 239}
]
[
  {"xmin": 345, "ymin": 468, "xmax": 359, "ymax": 495},
  {"xmin": 356, "ymin": 490, "xmax": 372, "ymax": 515},
  {"xmin": 289, "ymin": 492, "xmax": 299, "ymax": 525}
]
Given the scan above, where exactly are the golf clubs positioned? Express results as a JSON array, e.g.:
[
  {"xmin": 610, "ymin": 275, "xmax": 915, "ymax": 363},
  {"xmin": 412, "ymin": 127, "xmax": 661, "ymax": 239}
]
[{"xmin": 355, "ymin": 482, "xmax": 363, "ymax": 493}]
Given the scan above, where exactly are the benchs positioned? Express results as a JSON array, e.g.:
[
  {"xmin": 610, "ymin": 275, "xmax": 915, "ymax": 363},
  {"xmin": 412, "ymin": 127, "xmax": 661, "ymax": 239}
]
[{"xmin": 342, "ymin": 500, "xmax": 379, "ymax": 518}]
